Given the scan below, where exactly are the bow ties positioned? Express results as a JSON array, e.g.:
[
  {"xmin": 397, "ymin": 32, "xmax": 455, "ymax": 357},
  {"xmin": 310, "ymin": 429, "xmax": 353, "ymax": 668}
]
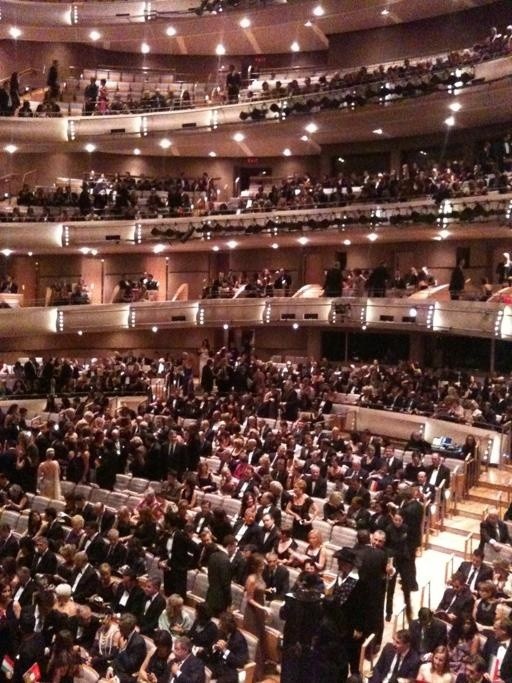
[{"xmin": 496, "ymin": 642, "xmax": 507, "ymax": 648}]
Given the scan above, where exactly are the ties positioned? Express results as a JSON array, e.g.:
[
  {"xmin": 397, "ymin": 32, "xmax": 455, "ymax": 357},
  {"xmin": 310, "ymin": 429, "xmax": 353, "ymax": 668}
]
[
  {"xmin": 392, "ymin": 653, "xmax": 401, "ymax": 673},
  {"xmin": 466, "ymin": 568, "xmax": 477, "ymax": 586}
]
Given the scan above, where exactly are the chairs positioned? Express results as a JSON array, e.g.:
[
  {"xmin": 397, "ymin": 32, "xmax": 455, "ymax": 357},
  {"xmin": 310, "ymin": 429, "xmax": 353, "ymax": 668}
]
[{"xmin": 1, "ymin": 417, "xmax": 512, "ymax": 683}]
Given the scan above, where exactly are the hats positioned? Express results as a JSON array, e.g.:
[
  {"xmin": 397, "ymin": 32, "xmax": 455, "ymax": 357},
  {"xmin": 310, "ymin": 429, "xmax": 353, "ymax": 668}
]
[{"xmin": 332, "ymin": 547, "xmax": 364, "ymax": 568}]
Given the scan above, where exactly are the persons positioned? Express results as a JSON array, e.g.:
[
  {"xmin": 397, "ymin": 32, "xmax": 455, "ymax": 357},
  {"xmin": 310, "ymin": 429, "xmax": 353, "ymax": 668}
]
[
  {"xmin": 0, "ymin": 341, "xmax": 512, "ymax": 683},
  {"xmin": 449, "ymin": 251, "xmax": 512, "ymax": 303},
  {"xmin": 246, "ymin": 25, "xmax": 512, "ymax": 102},
  {"xmin": 224, "ymin": 64, "xmax": 242, "ymax": 104},
  {"xmin": 0, "ymin": 142, "xmax": 507, "ymax": 223},
  {"xmin": 48, "ymin": 274, "xmax": 90, "ymax": 306},
  {"xmin": 0, "ymin": 272, "xmax": 20, "ymax": 293},
  {"xmin": 116, "ymin": 272, "xmax": 159, "ymax": 304},
  {"xmin": 204, "ymin": 267, "xmax": 291, "ymax": 299},
  {"xmin": 322, "ymin": 260, "xmax": 435, "ymax": 301},
  {"xmin": 0, "ymin": 59, "xmax": 192, "ymax": 118}
]
